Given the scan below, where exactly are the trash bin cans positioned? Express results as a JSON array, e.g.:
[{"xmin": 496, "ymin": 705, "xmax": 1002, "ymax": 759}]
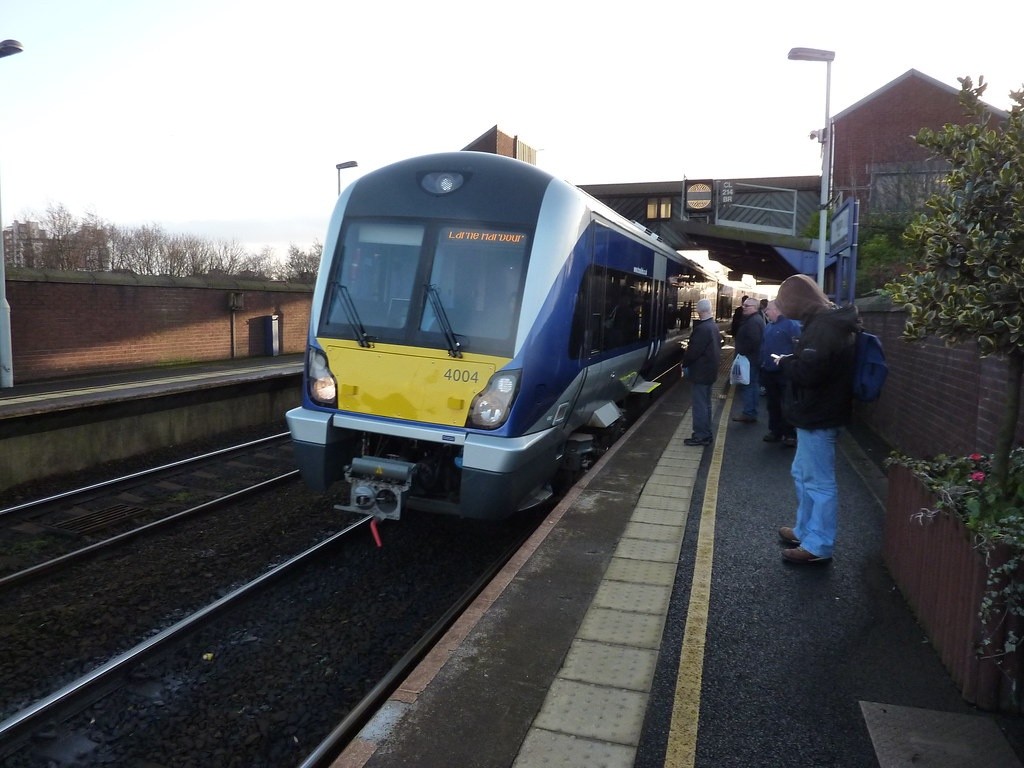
[{"xmin": 264, "ymin": 314, "xmax": 279, "ymax": 356}]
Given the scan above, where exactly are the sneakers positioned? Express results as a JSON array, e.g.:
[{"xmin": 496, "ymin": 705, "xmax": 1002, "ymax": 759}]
[{"xmin": 778, "ymin": 527, "xmax": 801, "ymax": 545}]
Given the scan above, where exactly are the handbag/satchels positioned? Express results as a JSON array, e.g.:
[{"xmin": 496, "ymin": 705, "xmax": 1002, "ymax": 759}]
[{"xmin": 730, "ymin": 353, "xmax": 751, "ymax": 386}]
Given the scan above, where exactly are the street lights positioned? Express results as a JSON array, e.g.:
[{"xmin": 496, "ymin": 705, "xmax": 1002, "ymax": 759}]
[
  {"xmin": 0, "ymin": 37, "xmax": 22, "ymax": 388},
  {"xmin": 787, "ymin": 47, "xmax": 835, "ymax": 295},
  {"xmin": 336, "ymin": 161, "xmax": 358, "ymax": 198}
]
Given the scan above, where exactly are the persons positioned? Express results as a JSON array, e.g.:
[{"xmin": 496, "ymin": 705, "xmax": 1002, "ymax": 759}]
[
  {"xmin": 680, "ymin": 299, "xmax": 720, "ymax": 446},
  {"xmin": 730, "ymin": 295, "xmax": 802, "ymax": 449},
  {"xmin": 776, "ymin": 274, "xmax": 863, "ymax": 564}
]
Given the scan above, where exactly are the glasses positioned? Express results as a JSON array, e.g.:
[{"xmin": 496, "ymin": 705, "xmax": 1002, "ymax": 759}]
[{"xmin": 742, "ymin": 304, "xmax": 756, "ymax": 309}]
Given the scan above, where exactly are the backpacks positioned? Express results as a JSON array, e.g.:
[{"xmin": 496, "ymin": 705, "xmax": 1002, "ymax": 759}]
[{"xmin": 843, "ymin": 330, "xmax": 888, "ymax": 414}]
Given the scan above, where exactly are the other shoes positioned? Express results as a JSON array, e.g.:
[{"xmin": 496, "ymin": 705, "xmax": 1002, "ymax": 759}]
[
  {"xmin": 781, "ymin": 545, "xmax": 832, "ymax": 564},
  {"xmin": 763, "ymin": 431, "xmax": 780, "ymax": 442},
  {"xmin": 683, "ymin": 433, "xmax": 712, "ymax": 445},
  {"xmin": 780, "ymin": 434, "xmax": 797, "ymax": 446},
  {"xmin": 732, "ymin": 414, "xmax": 757, "ymax": 423}
]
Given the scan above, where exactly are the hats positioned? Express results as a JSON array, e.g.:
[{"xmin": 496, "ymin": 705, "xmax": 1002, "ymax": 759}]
[{"xmin": 760, "ymin": 299, "xmax": 768, "ymax": 307}]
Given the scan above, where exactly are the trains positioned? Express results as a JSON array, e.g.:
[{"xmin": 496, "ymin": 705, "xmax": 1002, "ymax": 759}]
[{"xmin": 283, "ymin": 150, "xmax": 769, "ymax": 532}]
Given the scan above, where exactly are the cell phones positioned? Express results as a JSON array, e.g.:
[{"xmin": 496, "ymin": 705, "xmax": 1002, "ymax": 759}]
[{"xmin": 770, "ymin": 354, "xmax": 780, "ymax": 359}]
